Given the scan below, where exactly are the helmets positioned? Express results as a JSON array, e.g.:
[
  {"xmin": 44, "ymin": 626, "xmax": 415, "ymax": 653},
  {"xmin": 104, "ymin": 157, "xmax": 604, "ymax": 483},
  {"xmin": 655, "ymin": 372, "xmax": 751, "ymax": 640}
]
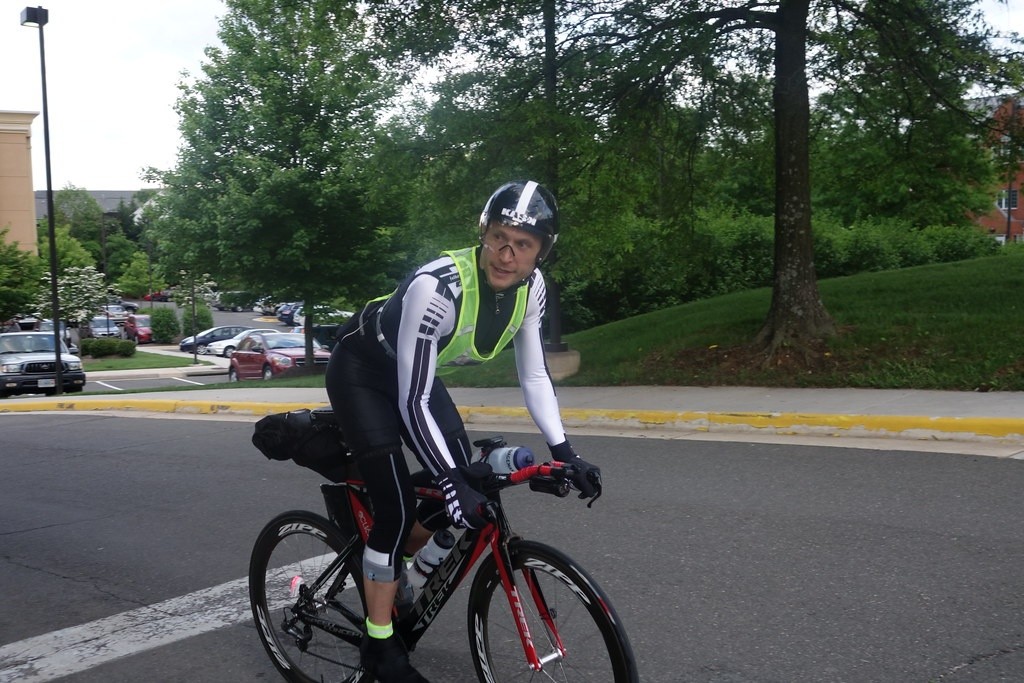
[{"xmin": 478, "ymin": 180, "xmax": 561, "ymax": 266}]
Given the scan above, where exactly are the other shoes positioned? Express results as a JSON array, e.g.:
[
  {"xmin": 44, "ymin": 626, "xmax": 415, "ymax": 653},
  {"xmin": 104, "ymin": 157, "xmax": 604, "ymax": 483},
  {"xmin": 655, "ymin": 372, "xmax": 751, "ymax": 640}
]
[{"xmin": 357, "ymin": 629, "xmax": 430, "ymax": 683}]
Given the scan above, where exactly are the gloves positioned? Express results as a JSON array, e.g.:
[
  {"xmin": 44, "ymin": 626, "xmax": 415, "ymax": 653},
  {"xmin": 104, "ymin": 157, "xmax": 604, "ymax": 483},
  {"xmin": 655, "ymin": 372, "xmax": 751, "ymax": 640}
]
[
  {"xmin": 433, "ymin": 468, "xmax": 489, "ymax": 531},
  {"xmin": 548, "ymin": 433, "xmax": 603, "ymax": 497}
]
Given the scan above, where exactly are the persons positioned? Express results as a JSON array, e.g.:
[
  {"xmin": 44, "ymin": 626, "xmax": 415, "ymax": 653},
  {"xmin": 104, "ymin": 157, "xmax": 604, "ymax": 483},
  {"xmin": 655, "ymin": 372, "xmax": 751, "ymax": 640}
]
[{"xmin": 324, "ymin": 179, "xmax": 601, "ymax": 683}]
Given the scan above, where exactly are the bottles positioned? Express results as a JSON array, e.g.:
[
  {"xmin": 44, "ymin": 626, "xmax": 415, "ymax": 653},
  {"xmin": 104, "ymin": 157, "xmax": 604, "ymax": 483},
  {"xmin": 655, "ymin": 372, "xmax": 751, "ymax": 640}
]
[
  {"xmin": 471, "ymin": 445, "xmax": 535, "ymax": 473},
  {"xmin": 406, "ymin": 528, "xmax": 455, "ymax": 604}
]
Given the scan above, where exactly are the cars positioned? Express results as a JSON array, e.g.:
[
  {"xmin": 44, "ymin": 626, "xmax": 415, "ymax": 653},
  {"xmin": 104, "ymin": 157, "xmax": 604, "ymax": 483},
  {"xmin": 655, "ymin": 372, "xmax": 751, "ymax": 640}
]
[
  {"xmin": 207, "ymin": 328, "xmax": 283, "ymax": 357},
  {"xmin": 180, "ymin": 326, "xmax": 255, "ymax": 355},
  {"xmin": 0, "ymin": 287, "xmax": 179, "ymax": 348},
  {"xmin": 0, "ymin": 332, "xmax": 87, "ymax": 397},
  {"xmin": 228, "ymin": 333, "xmax": 332, "ymax": 382},
  {"xmin": 204, "ymin": 284, "xmax": 359, "ymax": 326}
]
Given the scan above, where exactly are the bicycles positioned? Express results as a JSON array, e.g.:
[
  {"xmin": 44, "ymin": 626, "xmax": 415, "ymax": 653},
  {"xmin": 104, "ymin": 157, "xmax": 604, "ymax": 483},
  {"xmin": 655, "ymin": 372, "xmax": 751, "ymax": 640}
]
[{"xmin": 249, "ymin": 407, "xmax": 639, "ymax": 683}]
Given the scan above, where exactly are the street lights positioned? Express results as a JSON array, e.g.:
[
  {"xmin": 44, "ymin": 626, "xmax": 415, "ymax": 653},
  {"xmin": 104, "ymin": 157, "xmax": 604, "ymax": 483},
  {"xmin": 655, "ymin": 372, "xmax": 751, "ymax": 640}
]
[{"xmin": 20, "ymin": 5, "xmax": 64, "ymax": 399}]
